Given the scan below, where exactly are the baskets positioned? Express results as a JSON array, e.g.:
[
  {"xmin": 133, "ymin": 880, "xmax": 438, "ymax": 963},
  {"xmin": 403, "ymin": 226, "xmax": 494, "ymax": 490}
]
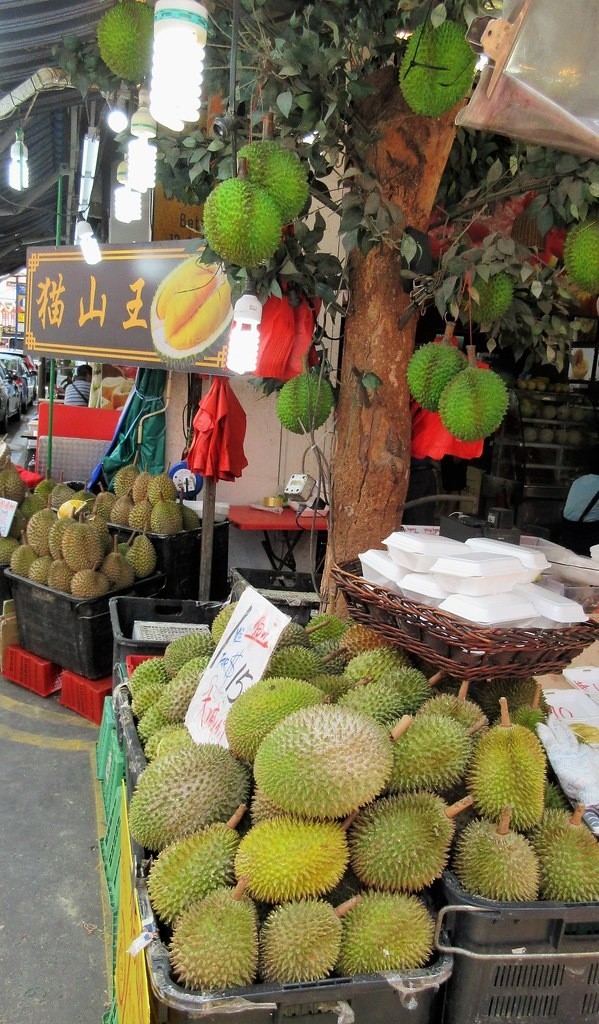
[{"xmin": 330, "ymin": 552, "xmax": 599, "ymax": 680}]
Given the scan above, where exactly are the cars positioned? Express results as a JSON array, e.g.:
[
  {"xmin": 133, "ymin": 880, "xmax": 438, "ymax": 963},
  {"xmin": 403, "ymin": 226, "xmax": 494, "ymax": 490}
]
[{"xmin": 0, "ymin": 347, "xmax": 57, "ymax": 434}]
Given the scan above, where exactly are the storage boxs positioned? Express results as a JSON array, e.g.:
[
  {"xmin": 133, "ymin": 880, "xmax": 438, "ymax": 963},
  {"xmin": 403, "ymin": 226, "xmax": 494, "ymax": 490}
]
[{"xmin": 0, "ymin": 517, "xmax": 598, "ymax": 1024}]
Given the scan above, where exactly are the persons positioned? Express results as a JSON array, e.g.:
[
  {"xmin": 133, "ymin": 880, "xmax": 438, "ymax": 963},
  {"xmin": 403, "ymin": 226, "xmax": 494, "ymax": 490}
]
[
  {"xmin": 435, "ymin": 452, "xmax": 467, "ymax": 515},
  {"xmin": 63, "ymin": 365, "xmax": 93, "ymax": 406},
  {"xmin": 562, "ymin": 445, "xmax": 599, "ymax": 548}
]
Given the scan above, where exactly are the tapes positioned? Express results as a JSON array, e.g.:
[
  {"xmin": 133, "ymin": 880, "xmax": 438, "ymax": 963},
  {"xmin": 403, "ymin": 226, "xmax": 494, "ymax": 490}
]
[{"xmin": 263, "ymin": 497, "xmax": 283, "ymax": 507}]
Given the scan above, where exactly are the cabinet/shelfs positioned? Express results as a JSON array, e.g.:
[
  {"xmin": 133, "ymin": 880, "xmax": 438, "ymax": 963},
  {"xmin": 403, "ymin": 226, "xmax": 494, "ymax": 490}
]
[{"xmin": 485, "ymin": 388, "xmax": 596, "ymax": 498}]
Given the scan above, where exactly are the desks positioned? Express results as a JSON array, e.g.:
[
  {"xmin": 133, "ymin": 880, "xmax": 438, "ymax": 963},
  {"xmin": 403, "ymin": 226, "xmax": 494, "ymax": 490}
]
[{"xmin": 226, "ymin": 505, "xmax": 330, "ymax": 572}]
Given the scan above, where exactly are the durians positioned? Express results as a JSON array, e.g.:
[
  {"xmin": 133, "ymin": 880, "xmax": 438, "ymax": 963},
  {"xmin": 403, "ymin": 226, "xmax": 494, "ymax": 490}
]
[
  {"xmin": 0, "ymin": 434, "xmax": 200, "ymax": 599},
  {"xmin": 125, "ymin": 599, "xmax": 599, "ymax": 989},
  {"xmin": 95, "ymin": 0, "xmax": 599, "ymax": 438}
]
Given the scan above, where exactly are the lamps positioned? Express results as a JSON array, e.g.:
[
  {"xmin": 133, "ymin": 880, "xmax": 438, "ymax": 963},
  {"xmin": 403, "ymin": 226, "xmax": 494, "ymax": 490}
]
[{"xmin": 8, "ymin": 0, "xmax": 212, "ymax": 264}]
